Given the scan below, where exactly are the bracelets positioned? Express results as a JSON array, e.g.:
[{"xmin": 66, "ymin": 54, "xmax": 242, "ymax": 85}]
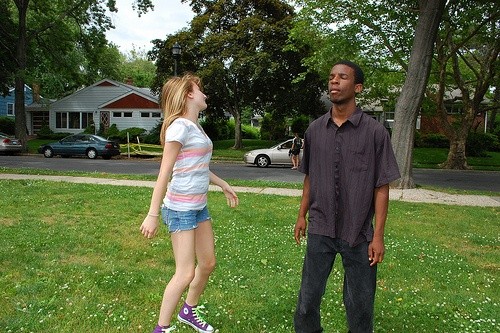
[{"xmin": 147, "ymin": 213, "xmax": 159, "ymax": 217}]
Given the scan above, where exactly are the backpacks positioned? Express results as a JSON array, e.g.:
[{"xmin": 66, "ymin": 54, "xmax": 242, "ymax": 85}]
[{"xmin": 295, "ymin": 137, "xmax": 302, "ymax": 152}]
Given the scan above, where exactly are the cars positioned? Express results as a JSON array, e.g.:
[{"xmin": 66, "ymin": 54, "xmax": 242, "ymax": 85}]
[
  {"xmin": 243, "ymin": 139, "xmax": 305, "ymax": 168},
  {"xmin": 0, "ymin": 132, "xmax": 12, "ymax": 139},
  {"xmin": 0, "ymin": 135, "xmax": 25, "ymax": 156},
  {"xmin": 38, "ymin": 133, "xmax": 121, "ymax": 159}
]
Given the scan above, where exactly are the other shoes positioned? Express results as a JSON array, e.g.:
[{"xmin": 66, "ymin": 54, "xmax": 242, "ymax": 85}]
[
  {"xmin": 177, "ymin": 303, "xmax": 215, "ymax": 333},
  {"xmin": 152, "ymin": 322, "xmax": 176, "ymax": 333},
  {"xmin": 291, "ymin": 167, "xmax": 297, "ymax": 170}
]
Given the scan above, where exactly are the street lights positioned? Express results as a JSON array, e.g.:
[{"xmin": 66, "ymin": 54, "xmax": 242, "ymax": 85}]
[{"xmin": 172, "ymin": 41, "xmax": 181, "ymax": 77}]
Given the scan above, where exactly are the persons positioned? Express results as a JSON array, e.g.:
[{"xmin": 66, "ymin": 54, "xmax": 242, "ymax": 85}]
[
  {"xmin": 89, "ymin": 119, "xmax": 96, "ymax": 135},
  {"xmin": 97, "ymin": 123, "xmax": 105, "ymax": 138},
  {"xmin": 290, "ymin": 132, "xmax": 304, "ymax": 170},
  {"xmin": 294, "ymin": 60, "xmax": 401, "ymax": 333},
  {"xmin": 139, "ymin": 73, "xmax": 239, "ymax": 333}
]
